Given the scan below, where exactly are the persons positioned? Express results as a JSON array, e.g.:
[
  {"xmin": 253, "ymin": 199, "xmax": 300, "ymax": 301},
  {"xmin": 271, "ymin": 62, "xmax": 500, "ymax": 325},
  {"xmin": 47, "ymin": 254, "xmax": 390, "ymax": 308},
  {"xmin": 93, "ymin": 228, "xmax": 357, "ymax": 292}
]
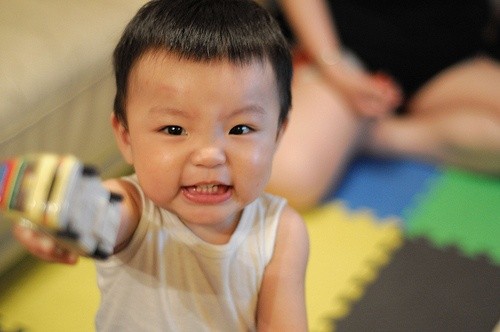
[
  {"xmin": 14, "ymin": 0, "xmax": 310, "ymax": 332},
  {"xmin": 266, "ymin": 0, "xmax": 500, "ymax": 212}
]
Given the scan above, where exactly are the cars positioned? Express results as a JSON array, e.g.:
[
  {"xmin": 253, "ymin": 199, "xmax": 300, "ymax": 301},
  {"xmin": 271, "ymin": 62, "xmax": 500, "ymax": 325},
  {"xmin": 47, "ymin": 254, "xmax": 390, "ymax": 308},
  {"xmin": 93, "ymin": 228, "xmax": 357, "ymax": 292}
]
[{"xmin": 0, "ymin": 153, "xmax": 123, "ymax": 261}]
